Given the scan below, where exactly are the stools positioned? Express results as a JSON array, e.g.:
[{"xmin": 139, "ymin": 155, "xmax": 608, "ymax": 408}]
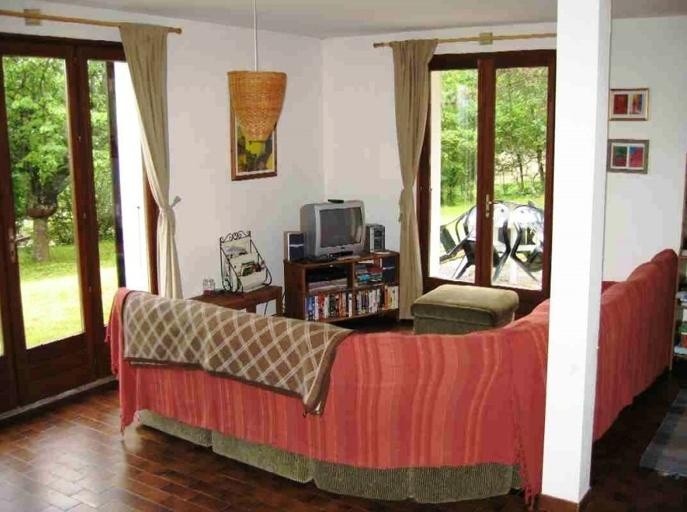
[{"xmin": 411, "ymin": 283, "xmax": 521, "ymax": 334}]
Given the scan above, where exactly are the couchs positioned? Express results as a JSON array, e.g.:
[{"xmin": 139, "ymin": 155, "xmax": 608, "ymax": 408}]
[{"xmin": 110, "ymin": 249, "xmax": 679, "ymax": 504}]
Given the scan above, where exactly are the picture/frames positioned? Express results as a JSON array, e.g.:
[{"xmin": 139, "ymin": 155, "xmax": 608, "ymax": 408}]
[
  {"xmin": 230, "ymin": 102, "xmax": 278, "ymax": 182},
  {"xmin": 606, "ymin": 88, "xmax": 650, "ymax": 174}
]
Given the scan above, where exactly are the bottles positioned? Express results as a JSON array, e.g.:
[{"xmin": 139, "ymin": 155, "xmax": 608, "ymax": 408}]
[{"xmin": 203, "ymin": 279, "xmax": 217, "ymax": 299}]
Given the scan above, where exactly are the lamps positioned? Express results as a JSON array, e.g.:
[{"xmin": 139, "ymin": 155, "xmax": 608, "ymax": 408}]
[{"xmin": 227, "ymin": 1, "xmax": 289, "ymax": 145}]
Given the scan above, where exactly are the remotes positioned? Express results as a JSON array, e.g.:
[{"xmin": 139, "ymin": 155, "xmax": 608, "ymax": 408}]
[{"xmin": 327, "ymin": 199, "xmax": 344, "ymax": 203}]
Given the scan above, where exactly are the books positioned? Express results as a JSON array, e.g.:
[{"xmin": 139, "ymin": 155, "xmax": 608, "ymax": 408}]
[{"xmin": 304, "ymin": 258, "xmax": 399, "ymax": 321}]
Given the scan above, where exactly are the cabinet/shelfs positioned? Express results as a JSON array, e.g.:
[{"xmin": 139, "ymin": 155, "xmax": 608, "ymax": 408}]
[
  {"xmin": 284, "ymin": 249, "xmax": 400, "ymax": 323},
  {"xmin": 191, "ymin": 285, "xmax": 282, "ymax": 317}
]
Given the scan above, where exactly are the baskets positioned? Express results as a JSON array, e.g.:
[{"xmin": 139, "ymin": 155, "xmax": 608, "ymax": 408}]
[{"xmin": 227, "ymin": 71, "xmax": 287, "ymax": 142}]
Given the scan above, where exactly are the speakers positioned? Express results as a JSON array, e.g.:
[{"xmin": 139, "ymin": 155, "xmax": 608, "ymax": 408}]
[
  {"xmin": 284, "ymin": 231, "xmax": 304, "ymax": 262},
  {"xmin": 366, "ymin": 224, "xmax": 386, "ymax": 254}
]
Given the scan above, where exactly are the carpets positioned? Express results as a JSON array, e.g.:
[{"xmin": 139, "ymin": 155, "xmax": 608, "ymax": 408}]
[{"xmin": 639, "ymin": 387, "xmax": 686, "ymax": 479}]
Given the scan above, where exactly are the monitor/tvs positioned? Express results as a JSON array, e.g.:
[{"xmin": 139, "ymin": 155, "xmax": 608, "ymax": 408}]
[{"xmin": 300, "ymin": 200, "xmax": 366, "ymax": 262}]
